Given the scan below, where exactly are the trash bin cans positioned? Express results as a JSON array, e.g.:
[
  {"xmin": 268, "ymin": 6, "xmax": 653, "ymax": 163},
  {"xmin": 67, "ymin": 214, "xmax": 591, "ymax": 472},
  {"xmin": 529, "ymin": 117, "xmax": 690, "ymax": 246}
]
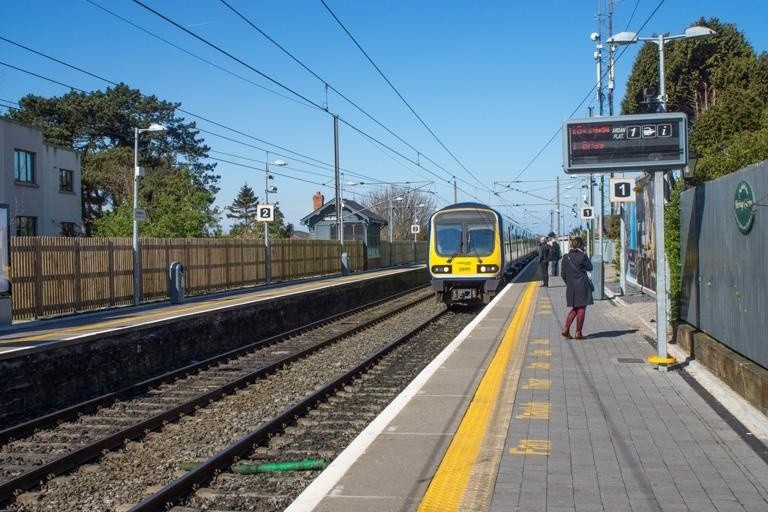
[
  {"xmin": 592, "ymin": 256, "xmax": 604, "ymax": 301},
  {"xmin": 169, "ymin": 261, "xmax": 186, "ymax": 304}
]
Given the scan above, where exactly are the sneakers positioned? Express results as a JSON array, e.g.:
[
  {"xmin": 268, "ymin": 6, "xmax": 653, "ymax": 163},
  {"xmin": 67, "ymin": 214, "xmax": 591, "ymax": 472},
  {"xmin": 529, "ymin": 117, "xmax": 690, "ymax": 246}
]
[
  {"xmin": 575, "ymin": 331, "xmax": 587, "ymax": 340},
  {"xmin": 561, "ymin": 328, "xmax": 572, "ymax": 339},
  {"xmin": 540, "ymin": 284, "xmax": 548, "ymax": 287}
]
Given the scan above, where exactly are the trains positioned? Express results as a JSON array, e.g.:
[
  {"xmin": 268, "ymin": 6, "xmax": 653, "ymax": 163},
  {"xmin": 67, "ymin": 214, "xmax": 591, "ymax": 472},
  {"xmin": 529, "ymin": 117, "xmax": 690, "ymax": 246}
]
[{"xmin": 427, "ymin": 202, "xmax": 539, "ymax": 308}]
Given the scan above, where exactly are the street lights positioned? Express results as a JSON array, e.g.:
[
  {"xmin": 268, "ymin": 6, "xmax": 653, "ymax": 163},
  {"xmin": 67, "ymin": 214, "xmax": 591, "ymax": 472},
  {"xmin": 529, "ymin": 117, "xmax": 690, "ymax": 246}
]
[
  {"xmin": 390, "ymin": 197, "xmax": 404, "ymax": 266},
  {"xmin": 563, "ymin": 174, "xmax": 594, "ymax": 261},
  {"xmin": 265, "ymin": 160, "xmax": 289, "ymax": 285},
  {"xmin": 133, "ymin": 122, "xmax": 170, "ymax": 305},
  {"xmin": 340, "ymin": 181, "xmax": 357, "ymax": 275},
  {"xmin": 605, "ymin": 26, "xmax": 720, "ymax": 373},
  {"xmin": 414, "ymin": 204, "xmax": 426, "ymax": 264}
]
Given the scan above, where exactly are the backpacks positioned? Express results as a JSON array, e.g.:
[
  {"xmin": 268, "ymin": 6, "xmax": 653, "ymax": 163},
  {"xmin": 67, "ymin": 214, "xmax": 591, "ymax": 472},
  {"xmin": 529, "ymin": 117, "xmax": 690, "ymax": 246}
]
[{"xmin": 542, "ymin": 245, "xmax": 558, "ymax": 261}]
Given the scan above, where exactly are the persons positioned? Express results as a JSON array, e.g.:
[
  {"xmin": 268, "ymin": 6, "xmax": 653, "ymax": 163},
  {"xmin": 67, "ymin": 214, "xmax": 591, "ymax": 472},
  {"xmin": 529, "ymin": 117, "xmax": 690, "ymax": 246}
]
[
  {"xmin": 539, "ymin": 236, "xmax": 552, "ymax": 287},
  {"xmin": 561, "ymin": 236, "xmax": 596, "ymax": 339},
  {"xmin": 548, "ymin": 237, "xmax": 562, "ymax": 276}
]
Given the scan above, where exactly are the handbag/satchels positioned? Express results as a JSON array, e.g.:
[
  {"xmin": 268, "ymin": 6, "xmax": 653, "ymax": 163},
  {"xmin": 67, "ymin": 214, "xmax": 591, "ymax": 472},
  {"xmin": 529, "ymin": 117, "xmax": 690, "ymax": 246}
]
[{"xmin": 589, "ymin": 279, "xmax": 594, "ymax": 291}]
